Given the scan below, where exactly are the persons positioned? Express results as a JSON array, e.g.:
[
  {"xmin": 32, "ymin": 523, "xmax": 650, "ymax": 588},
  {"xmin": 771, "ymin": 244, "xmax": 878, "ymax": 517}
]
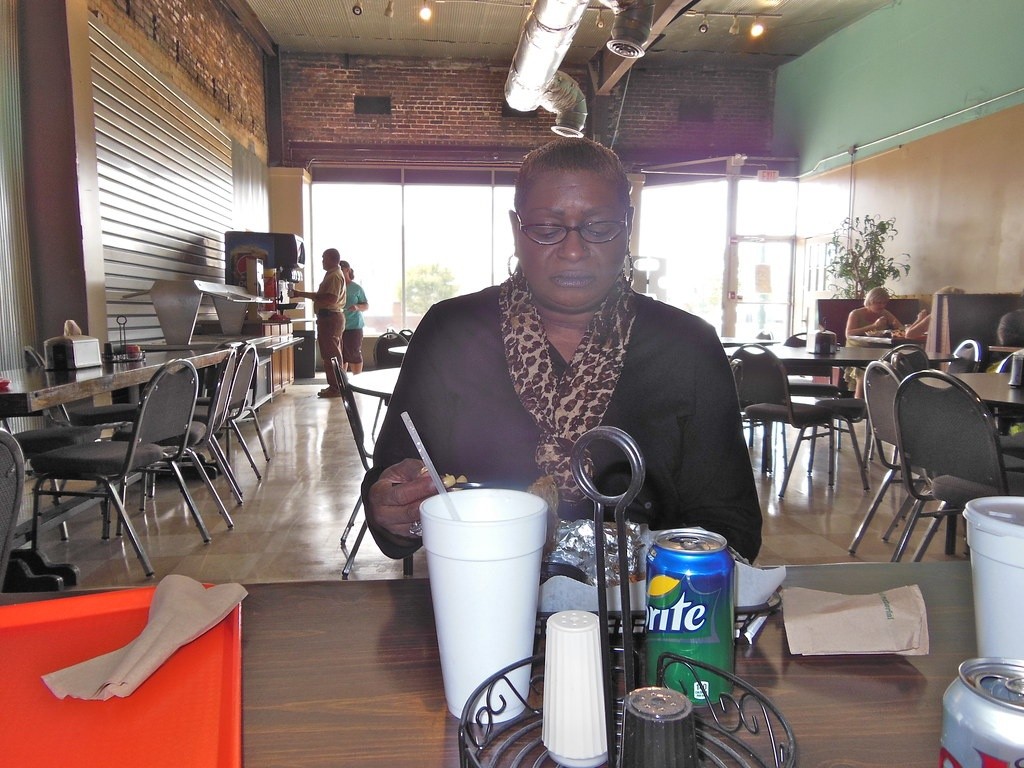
[
  {"xmin": 287, "ymin": 248, "xmax": 345, "ymax": 398},
  {"xmin": 906, "ymin": 286, "xmax": 966, "ymax": 340},
  {"xmin": 338, "ymin": 261, "xmax": 369, "ymax": 375},
  {"xmin": 359, "ymin": 142, "xmax": 764, "ymax": 566},
  {"xmin": 844, "ymin": 288, "xmax": 906, "ymax": 400},
  {"xmin": 982, "ymin": 309, "xmax": 1024, "ymax": 434}
]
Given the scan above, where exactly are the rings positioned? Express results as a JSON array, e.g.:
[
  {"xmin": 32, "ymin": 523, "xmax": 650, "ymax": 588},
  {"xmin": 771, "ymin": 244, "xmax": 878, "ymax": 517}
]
[{"xmin": 409, "ymin": 521, "xmax": 423, "ymax": 536}]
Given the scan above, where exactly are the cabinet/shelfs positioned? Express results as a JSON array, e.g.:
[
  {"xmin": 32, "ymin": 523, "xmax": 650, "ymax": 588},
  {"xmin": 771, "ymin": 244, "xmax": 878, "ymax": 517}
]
[{"xmin": 202, "ymin": 324, "xmax": 294, "ymax": 396}]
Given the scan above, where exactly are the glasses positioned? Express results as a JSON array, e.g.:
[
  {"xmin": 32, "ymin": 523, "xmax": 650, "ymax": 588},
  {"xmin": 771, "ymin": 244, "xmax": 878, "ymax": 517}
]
[{"xmin": 513, "ymin": 211, "xmax": 630, "ymax": 246}]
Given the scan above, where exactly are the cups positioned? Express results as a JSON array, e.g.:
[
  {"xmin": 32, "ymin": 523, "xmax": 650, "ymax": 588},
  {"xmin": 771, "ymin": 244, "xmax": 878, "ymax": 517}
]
[
  {"xmin": 963, "ymin": 494, "xmax": 1023, "ymax": 662},
  {"xmin": 418, "ymin": 488, "xmax": 548, "ymax": 725}
]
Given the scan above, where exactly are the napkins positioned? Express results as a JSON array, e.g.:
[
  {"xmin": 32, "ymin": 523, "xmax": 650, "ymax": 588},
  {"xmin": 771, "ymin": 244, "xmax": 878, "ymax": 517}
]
[{"xmin": 40, "ymin": 573, "xmax": 250, "ymax": 701}]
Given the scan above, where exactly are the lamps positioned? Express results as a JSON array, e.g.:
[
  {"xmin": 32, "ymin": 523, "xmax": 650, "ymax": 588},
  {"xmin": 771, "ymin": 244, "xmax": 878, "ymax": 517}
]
[
  {"xmin": 595, "ymin": 9, "xmax": 604, "ymax": 29},
  {"xmin": 732, "ymin": 153, "xmax": 748, "ymax": 166},
  {"xmin": 729, "ymin": 15, "xmax": 739, "ymax": 36},
  {"xmin": 384, "ymin": 0, "xmax": 394, "ymax": 18},
  {"xmin": 697, "ymin": 14, "xmax": 709, "ymax": 33},
  {"xmin": 352, "ymin": 0, "xmax": 363, "ymax": 15},
  {"xmin": 751, "ymin": 16, "xmax": 763, "ymax": 37}
]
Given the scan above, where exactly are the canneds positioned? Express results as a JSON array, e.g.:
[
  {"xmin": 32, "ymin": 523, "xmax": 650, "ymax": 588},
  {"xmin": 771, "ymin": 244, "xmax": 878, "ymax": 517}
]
[
  {"xmin": 938, "ymin": 656, "xmax": 1024, "ymax": 768},
  {"xmin": 641, "ymin": 527, "xmax": 733, "ymax": 706}
]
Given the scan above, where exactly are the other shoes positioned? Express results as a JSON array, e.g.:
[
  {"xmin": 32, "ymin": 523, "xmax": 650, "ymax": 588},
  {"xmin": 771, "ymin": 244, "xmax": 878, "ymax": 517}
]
[{"xmin": 318, "ymin": 385, "xmax": 342, "ymax": 397}]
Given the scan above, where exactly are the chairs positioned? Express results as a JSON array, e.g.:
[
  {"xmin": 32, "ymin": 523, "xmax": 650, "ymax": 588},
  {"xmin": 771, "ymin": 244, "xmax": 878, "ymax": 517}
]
[
  {"xmin": 330, "ymin": 356, "xmax": 374, "ymax": 576},
  {"xmin": 730, "ymin": 328, "xmax": 1024, "ymax": 562},
  {"xmin": 371, "ymin": 329, "xmax": 414, "ymax": 438},
  {"xmin": 0, "ymin": 342, "xmax": 271, "ymax": 590}
]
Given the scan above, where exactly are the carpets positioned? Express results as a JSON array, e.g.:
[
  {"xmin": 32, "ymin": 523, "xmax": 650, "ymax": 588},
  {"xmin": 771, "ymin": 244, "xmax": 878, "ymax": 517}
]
[{"xmin": 293, "ymin": 378, "xmax": 329, "ymax": 385}]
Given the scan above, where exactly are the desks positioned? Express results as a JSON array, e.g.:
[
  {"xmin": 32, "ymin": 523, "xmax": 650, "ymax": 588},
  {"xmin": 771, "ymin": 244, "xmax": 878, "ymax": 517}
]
[
  {"xmin": 0, "ymin": 560, "xmax": 979, "ymax": 768},
  {"xmin": 722, "ymin": 346, "xmax": 961, "ymax": 475},
  {"xmin": 849, "ymin": 336, "xmax": 927, "ymax": 344},
  {"xmin": 347, "ymin": 345, "xmax": 414, "ymax": 575},
  {"xmin": 988, "ymin": 345, "xmax": 1024, "ymax": 352},
  {"xmin": 0, "ymin": 347, "xmax": 238, "ymax": 587},
  {"xmin": 719, "ymin": 336, "xmax": 780, "ymax": 348},
  {"xmin": 919, "ymin": 373, "xmax": 1023, "ymax": 555}
]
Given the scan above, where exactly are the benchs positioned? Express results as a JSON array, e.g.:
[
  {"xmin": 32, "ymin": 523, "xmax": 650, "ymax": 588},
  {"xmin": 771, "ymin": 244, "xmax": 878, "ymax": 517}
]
[
  {"xmin": 924, "ymin": 293, "xmax": 1024, "ymax": 374},
  {"xmin": 813, "ymin": 299, "xmax": 919, "ymax": 388}
]
